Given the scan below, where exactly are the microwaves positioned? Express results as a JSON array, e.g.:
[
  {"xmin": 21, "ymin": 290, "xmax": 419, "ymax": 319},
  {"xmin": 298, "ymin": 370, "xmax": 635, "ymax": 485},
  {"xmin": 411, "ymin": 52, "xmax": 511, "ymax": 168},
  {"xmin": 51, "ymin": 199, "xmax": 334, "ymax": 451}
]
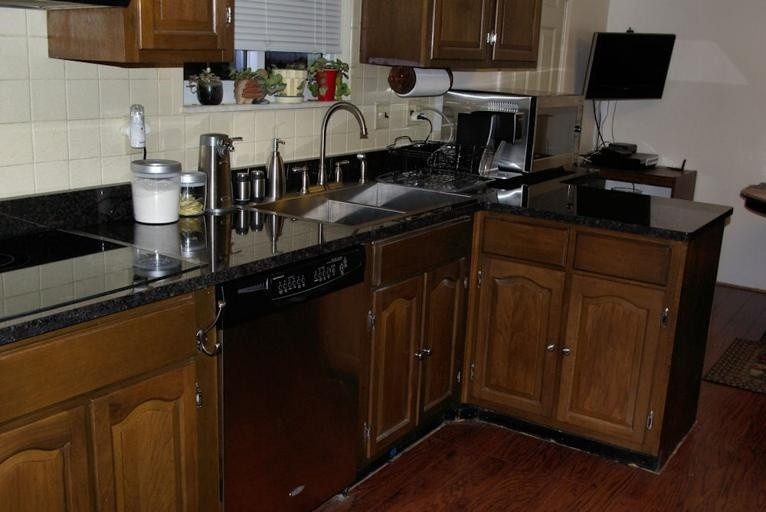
[{"xmin": 440, "ymin": 86, "xmax": 587, "ymax": 175}]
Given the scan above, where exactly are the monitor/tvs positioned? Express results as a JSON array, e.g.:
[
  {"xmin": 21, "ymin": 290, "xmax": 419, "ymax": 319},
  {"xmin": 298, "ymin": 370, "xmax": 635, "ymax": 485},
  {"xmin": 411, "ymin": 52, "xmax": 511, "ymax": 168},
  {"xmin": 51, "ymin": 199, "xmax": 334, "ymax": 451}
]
[{"xmin": 582, "ymin": 31, "xmax": 676, "ymax": 101}]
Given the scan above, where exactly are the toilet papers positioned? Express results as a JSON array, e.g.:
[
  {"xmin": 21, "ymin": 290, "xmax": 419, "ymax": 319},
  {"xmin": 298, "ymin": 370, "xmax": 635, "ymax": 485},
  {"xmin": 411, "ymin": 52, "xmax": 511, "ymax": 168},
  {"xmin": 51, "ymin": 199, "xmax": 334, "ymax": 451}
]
[{"xmin": 393, "ymin": 67, "xmax": 450, "ymax": 98}]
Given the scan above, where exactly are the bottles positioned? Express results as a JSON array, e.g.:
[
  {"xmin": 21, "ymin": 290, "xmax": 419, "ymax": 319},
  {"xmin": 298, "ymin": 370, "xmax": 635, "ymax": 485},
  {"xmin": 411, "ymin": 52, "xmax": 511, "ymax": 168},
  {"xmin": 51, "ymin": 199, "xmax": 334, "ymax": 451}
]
[
  {"xmin": 130, "ymin": 157, "xmax": 182, "ymax": 224},
  {"xmin": 199, "ymin": 133, "xmax": 266, "ymax": 216},
  {"xmin": 180, "ymin": 171, "xmax": 207, "ymax": 215}
]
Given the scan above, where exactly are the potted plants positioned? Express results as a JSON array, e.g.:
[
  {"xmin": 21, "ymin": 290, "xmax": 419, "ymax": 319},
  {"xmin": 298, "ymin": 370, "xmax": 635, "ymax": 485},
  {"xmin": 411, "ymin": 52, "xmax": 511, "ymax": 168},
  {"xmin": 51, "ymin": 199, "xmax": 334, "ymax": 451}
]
[{"xmin": 184, "ymin": 54, "xmax": 351, "ymax": 103}]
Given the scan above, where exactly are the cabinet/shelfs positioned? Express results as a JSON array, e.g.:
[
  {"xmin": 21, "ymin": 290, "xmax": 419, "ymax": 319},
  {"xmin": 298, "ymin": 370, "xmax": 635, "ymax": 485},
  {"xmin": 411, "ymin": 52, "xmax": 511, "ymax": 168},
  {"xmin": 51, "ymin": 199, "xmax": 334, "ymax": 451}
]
[
  {"xmin": 361, "ymin": 3, "xmax": 544, "ymax": 70},
  {"xmin": 288, "ymin": 211, "xmax": 472, "ymax": 460},
  {"xmin": 48, "ymin": 1, "xmax": 236, "ymax": 69},
  {"xmin": 0, "ymin": 296, "xmax": 204, "ymax": 512},
  {"xmin": 468, "ymin": 211, "xmax": 726, "ymax": 475}
]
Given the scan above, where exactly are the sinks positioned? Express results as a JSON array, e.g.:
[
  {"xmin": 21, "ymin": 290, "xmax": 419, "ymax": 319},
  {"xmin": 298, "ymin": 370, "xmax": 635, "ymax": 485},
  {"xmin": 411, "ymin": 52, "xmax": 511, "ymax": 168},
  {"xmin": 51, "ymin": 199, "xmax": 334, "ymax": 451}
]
[
  {"xmin": 232, "ymin": 193, "xmax": 407, "ymax": 229},
  {"xmin": 320, "ymin": 182, "xmax": 475, "ymax": 215}
]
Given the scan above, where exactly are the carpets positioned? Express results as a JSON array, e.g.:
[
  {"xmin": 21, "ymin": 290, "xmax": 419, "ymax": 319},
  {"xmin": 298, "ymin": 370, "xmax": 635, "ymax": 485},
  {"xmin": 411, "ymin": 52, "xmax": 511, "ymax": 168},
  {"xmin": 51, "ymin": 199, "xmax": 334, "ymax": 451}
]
[{"xmin": 700, "ymin": 337, "xmax": 764, "ymax": 394}]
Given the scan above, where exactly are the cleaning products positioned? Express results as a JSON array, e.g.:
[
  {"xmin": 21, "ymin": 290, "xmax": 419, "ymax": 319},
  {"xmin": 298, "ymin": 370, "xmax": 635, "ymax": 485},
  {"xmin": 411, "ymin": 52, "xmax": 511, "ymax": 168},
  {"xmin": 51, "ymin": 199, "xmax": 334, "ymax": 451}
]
[
  {"xmin": 264, "ymin": 213, "xmax": 287, "ymax": 255},
  {"xmin": 265, "ymin": 137, "xmax": 288, "ymax": 200}
]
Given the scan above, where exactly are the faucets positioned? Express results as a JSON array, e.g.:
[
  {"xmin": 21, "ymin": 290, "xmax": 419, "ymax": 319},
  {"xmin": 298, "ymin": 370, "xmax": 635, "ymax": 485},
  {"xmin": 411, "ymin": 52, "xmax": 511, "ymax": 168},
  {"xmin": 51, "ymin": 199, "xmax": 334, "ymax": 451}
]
[{"xmin": 316, "ymin": 101, "xmax": 368, "ymax": 187}]
